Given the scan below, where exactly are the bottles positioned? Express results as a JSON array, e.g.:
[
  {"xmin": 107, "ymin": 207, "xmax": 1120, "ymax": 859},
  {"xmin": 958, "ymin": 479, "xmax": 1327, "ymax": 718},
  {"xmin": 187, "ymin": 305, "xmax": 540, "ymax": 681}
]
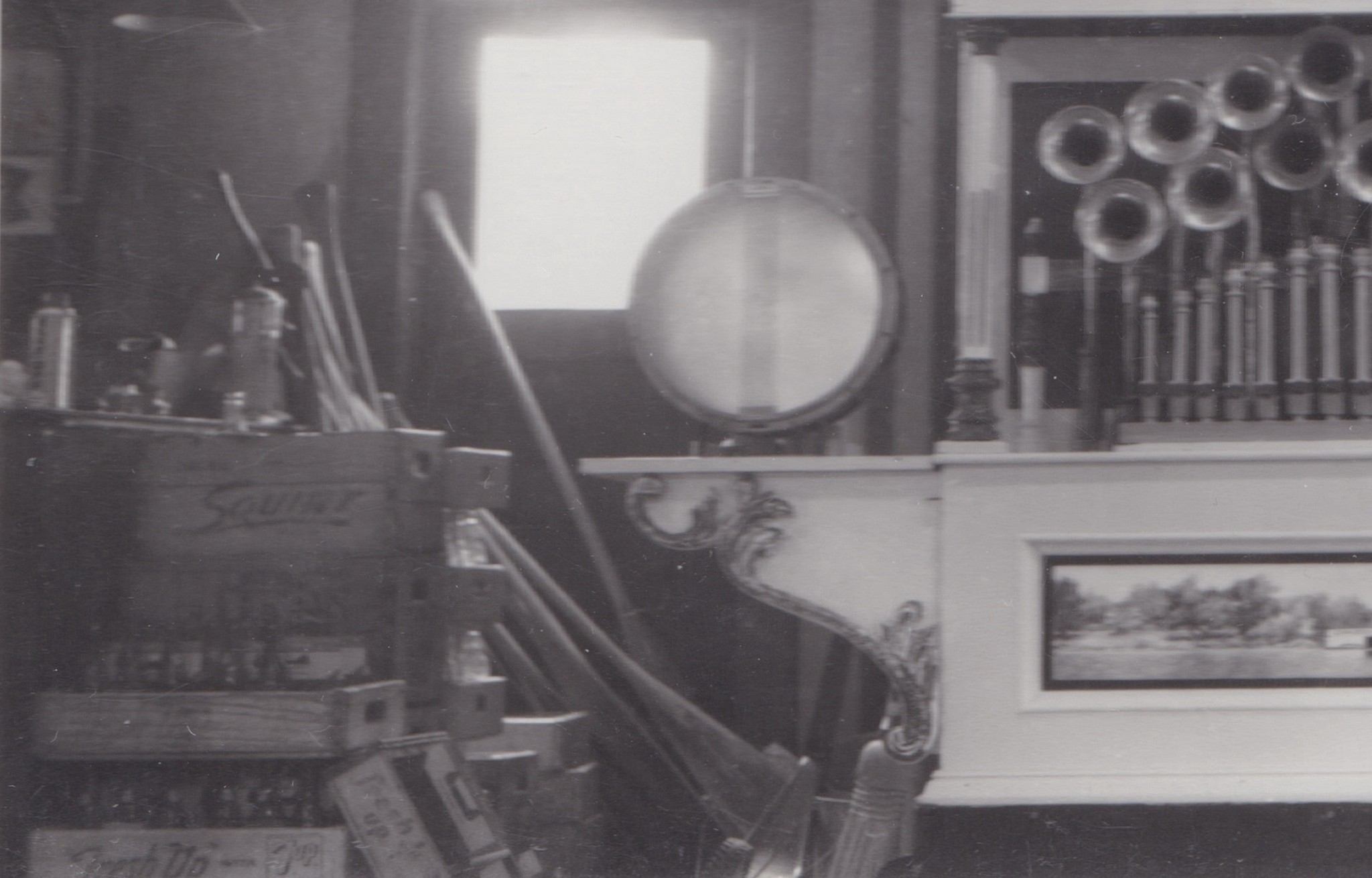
[{"xmin": 31, "ymin": 306, "xmax": 78, "ymax": 409}]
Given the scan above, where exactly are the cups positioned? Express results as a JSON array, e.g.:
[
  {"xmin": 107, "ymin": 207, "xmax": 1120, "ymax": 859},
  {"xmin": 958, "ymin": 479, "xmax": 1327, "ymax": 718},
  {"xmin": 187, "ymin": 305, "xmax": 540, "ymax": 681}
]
[{"xmin": 227, "ymin": 292, "xmax": 286, "ymax": 424}]
